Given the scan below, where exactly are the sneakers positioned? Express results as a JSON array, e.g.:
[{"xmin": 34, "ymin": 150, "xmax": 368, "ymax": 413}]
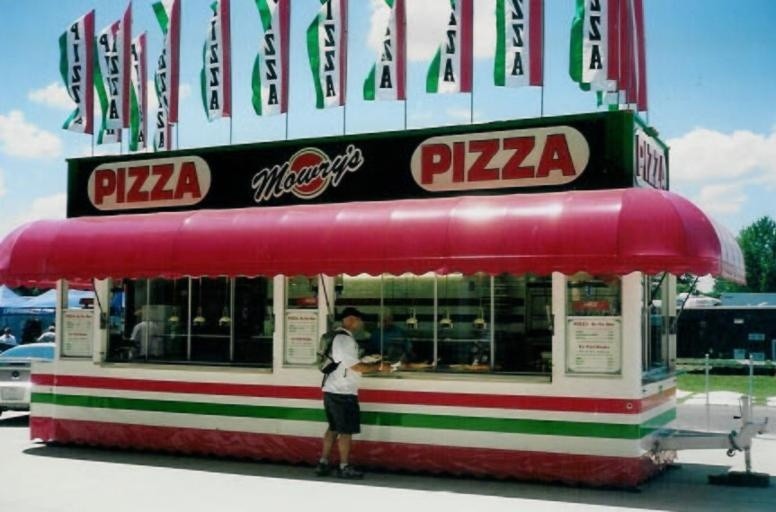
[
  {"xmin": 314, "ymin": 462, "xmax": 332, "ymax": 475},
  {"xmin": 338, "ymin": 465, "xmax": 362, "ymax": 478}
]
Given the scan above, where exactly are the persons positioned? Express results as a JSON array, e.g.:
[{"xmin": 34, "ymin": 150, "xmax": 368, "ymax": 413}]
[
  {"xmin": 366, "ymin": 313, "xmax": 417, "ymax": 370},
  {"xmin": 130, "ymin": 315, "xmax": 160, "ymax": 358},
  {"xmin": 314, "ymin": 307, "xmax": 391, "ymax": 477},
  {"xmin": 0, "ymin": 327, "xmax": 19, "ymax": 346},
  {"xmin": 43, "ymin": 322, "xmax": 55, "ymax": 331},
  {"xmin": 37, "ymin": 325, "xmax": 56, "ymax": 342}
]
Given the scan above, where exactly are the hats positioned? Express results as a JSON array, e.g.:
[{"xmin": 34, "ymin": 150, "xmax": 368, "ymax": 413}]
[{"xmin": 340, "ymin": 307, "xmax": 365, "ymax": 318}]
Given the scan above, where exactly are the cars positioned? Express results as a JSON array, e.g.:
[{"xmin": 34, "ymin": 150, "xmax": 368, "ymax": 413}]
[{"xmin": 0, "ymin": 341, "xmax": 55, "ymax": 416}]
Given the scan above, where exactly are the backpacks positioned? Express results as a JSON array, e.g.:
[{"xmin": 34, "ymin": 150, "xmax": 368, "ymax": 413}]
[{"xmin": 316, "ymin": 330, "xmax": 350, "ymax": 373}]
